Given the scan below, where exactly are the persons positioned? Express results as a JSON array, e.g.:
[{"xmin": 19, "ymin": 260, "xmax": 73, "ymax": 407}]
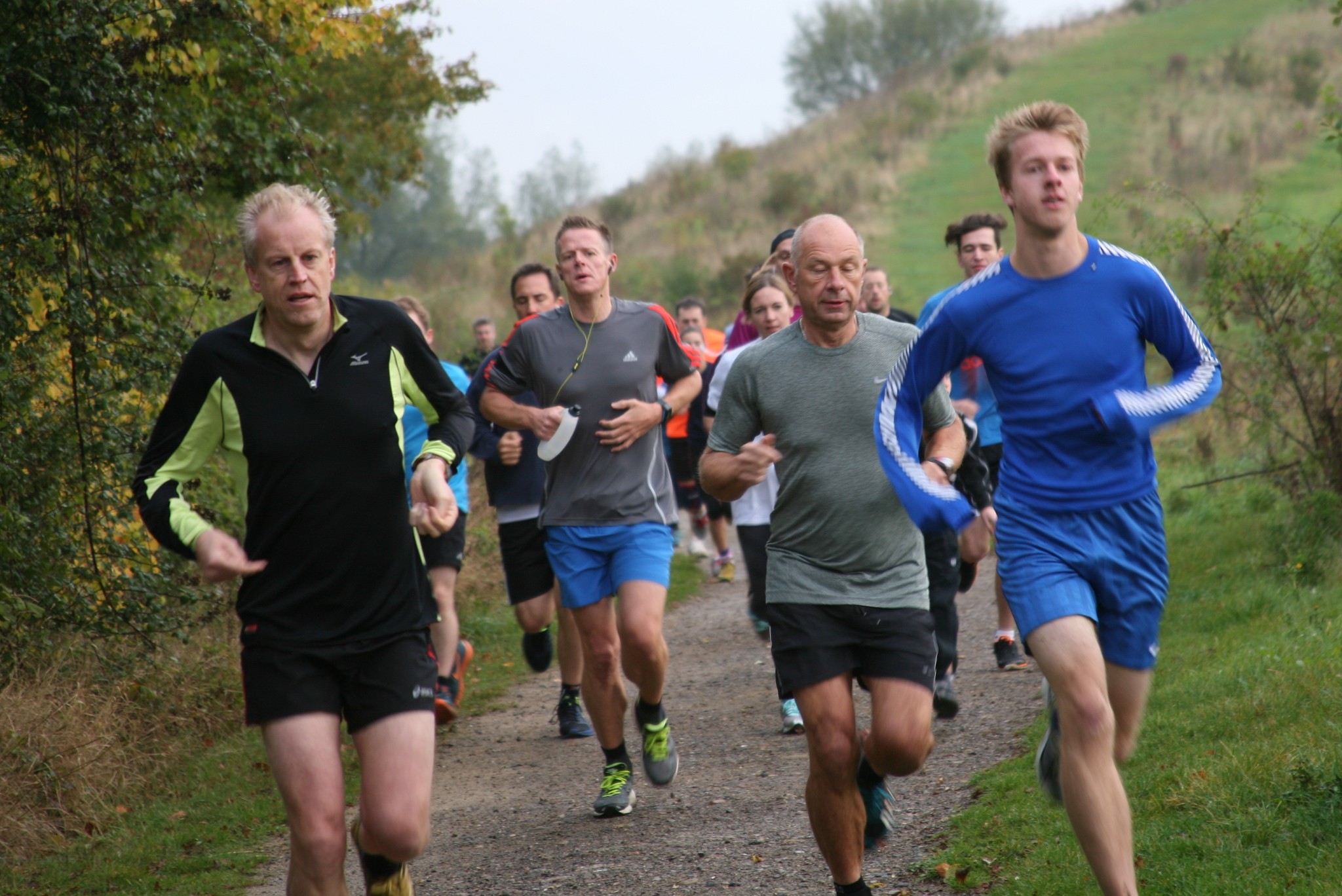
[
  {"xmin": 697, "ymin": 213, "xmax": 968, "ymax": 896},
  {"xmin": 483, "ymin": 215, "xmax": 705, "ymax": 823},
  {"xmin": 457, "ymin": 216, "xmax": 1007, "ymax": 738},
  {"xmin": 874, "ymin": 102, "xmax": 1221, "ymax": 896},
  {"xmin": 129, "ymin": 185, "xmax": 474, "ymax": 896},
  {"xmin": 391, "ymin": 297, "xmax": 473, "ymax": 721}
]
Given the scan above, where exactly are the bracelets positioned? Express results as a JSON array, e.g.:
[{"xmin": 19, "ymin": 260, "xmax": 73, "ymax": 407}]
[{"xmin": 412, "ymin": 453, "xmax": 452, "ymax": 482}]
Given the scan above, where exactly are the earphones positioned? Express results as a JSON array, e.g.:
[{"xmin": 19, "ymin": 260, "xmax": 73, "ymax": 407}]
[
  {"xmin": 608, "ymin": 263, "xmax": 614, "ymax": 275},
  {"xmin": 558, "ymin": 272, "xmax": 563, "ymax": 280}
]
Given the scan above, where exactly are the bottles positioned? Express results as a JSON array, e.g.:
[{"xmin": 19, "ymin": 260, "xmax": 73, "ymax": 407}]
[{"xmin": 538, "ymin": 404, "xmax": 581, "ymax": 462}]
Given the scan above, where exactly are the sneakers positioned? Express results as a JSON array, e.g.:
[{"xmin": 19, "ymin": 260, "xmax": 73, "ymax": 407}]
[
  {"xmin": 666, "ymin": 512, "xmax": 709, "ymax": 561},
  {"xmin": 351, "ymin": 813, "xmax": 414, "ymax": 896},
  {"xmin": 717, "ymin": 561, "xmax": 735, "ymax": 582},
  {"xmin": 434, "ymin": 637, "xmax": 474, "ymax": 706},
  {"xmin": 591, "ymin": 758, "xmax": 637, "ymax": 817},
  {"xmin": 932, "ymin": 676, "xmax": 959, "ymax": 711},
  {"xmin": 522, "ymin": 623, "xmax": 553, "ymax": 673},
  {"xmin": 1034, "ymin": 674, "xmax": 1064, "ymax": 803},
  {"xmin": 746, "ymin": 588, "xmax": 770, "ymax": 641},
  {"xmin": 631, "ymin": 691, "xmax": 679, "ymax": 785},
  {"xmin": 433, "ymin": 676, "xmax": 459, "ymax": 724},
  {"xmin": 557, "ymin": 688, "xmax": 594, "ymax": 737},
  {"xmin": 779, "ymin": 698, "xmax": 805, "ymax": 735},
  {"xmin": 856, "ymin": 727, "xmax": 897, "ymax": 849},
  {"xmin": 993, "ymin": 635, "xmax": 1027, "ymax": 671}
]
